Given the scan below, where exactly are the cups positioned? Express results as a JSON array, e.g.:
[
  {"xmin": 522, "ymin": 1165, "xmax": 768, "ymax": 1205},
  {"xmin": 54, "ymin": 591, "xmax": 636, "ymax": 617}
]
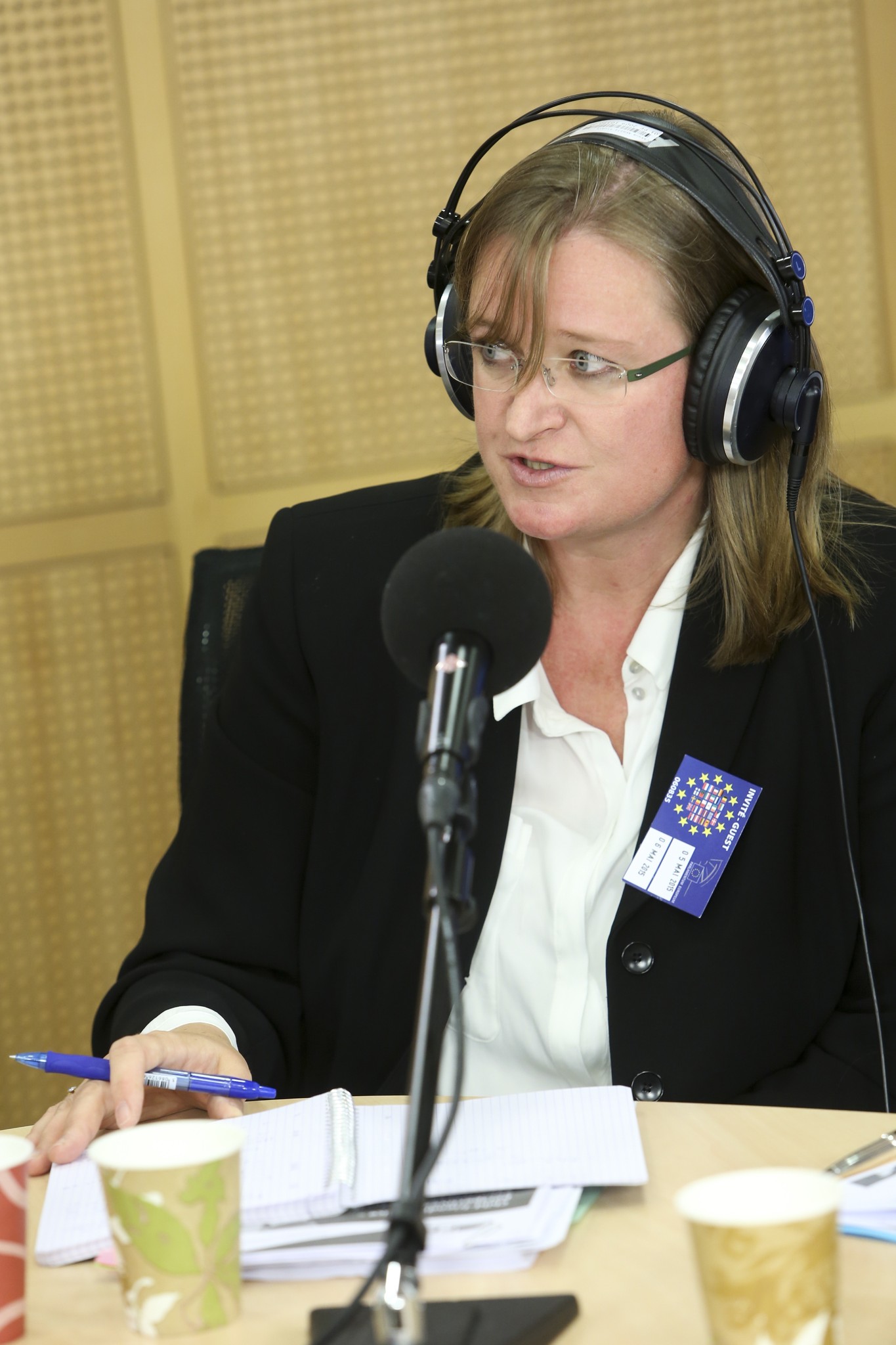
[
  {"xmin": 1, "ymin": 1130, "xmax": 37, "ymax": 1342},
  {"xmin": 676, "ymin": 1167, "xmax": 843, "ymax": 1345},
  {"xmin": 86, "ymin": 1113, "xmax": 243, "ymax": 1338}
]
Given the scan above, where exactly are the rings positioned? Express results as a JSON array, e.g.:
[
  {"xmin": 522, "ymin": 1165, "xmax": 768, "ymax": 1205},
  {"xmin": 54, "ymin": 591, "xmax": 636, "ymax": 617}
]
[{"xmin": 66, "ymin": 1085, "xmax": 77, "ymax": 1094}]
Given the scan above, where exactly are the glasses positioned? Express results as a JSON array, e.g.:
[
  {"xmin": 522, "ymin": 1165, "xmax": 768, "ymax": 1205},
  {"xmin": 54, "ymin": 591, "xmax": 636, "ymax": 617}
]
[{"xmin": 441, "ymin": 334, "xmax": 694, "ymax": 405}]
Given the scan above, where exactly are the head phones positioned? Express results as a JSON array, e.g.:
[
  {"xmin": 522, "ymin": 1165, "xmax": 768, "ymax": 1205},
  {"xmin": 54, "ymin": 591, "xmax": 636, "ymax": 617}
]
[{"xmin": 420, "ymin": 90, "xmax": 823, "ymax": 473}]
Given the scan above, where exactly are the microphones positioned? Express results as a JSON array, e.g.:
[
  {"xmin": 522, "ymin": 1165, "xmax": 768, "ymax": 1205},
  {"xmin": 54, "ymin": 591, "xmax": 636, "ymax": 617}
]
[{"xmin": 376, "ymin": 525, "xmax": 554, "ymax": 831}]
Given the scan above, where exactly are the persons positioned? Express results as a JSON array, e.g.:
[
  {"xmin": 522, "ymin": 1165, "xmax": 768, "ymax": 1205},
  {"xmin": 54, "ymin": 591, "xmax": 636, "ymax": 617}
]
[{"xmin": 19, "ymin": 106, "xmax": 896, "ymax": 1182}]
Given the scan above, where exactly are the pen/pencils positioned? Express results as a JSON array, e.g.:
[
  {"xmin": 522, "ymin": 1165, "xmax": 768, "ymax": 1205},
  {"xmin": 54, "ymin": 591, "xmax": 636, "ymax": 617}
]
[{"xmin": 9, "ymin": 1051, "xmax": 277, "ymax": 1101}]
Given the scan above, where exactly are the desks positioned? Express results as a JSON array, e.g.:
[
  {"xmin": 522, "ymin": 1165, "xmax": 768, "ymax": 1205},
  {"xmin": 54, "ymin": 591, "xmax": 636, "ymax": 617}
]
[{"xmin": 0, "ymin": 1090, "xmax": 896, "ymax": 1345}]
